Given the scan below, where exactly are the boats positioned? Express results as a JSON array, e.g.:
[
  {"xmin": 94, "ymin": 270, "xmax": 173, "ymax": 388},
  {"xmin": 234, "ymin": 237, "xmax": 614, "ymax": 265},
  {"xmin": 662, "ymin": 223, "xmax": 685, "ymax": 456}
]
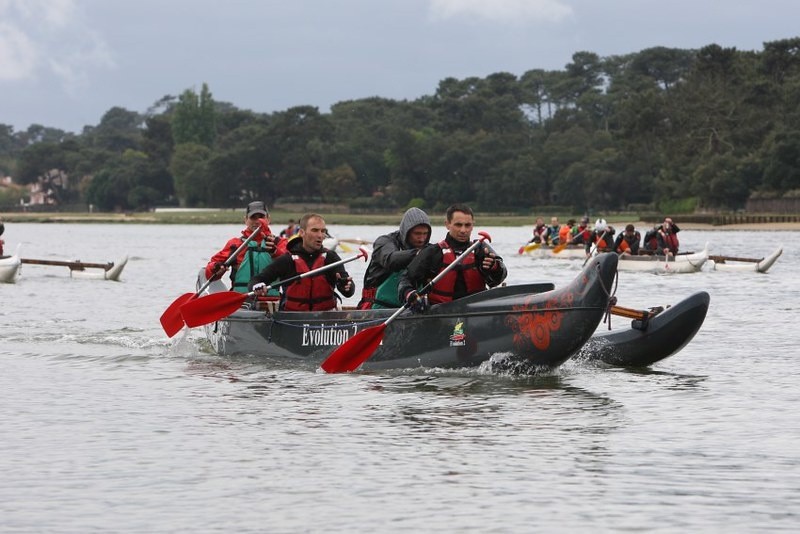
[
  {"xmin": 525, "ymin": 243, "xmax": 599, "ymax": 258},
  {"xmin": 0, "ymin": 252, "xmax": 25, "ymax": 284},
  {"xmin": 592, "ymin": 246, "xmax": 784, "ymax": 274},
  {"xmin": 196, "ymin": 266, "xmax": 307, "ymax": 390},
  {"xmin": 252, "ymin": 250, "xmax": 710, "ymax": 375}
]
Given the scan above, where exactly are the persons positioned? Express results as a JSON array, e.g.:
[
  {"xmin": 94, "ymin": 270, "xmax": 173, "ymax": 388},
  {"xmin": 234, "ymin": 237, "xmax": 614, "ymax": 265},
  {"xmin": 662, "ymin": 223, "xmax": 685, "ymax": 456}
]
[
  {"xmin": 399, "ymin": 202, "xmax": 507, "ymax": 314},
  {"xmin": 294, "ymin": 219, "xmax": 301, "ymax": 234},
  {"xmin": 358, "ymin": 208, "xmax": 431, "ymax": 309},
  {"xmin": 281, "ymin": 219, "xmax": 296, "ymax": 238},
  {"xmin": 533, "ymin": 216, "xmax": 680, "ymax": 257},
  {"xmin": 247, "ymin": 213, "xmax": 356, "ymax": 311},
  {"xmin": 206, "ymin": 201, "xmax": 288, "ymax": 310}
]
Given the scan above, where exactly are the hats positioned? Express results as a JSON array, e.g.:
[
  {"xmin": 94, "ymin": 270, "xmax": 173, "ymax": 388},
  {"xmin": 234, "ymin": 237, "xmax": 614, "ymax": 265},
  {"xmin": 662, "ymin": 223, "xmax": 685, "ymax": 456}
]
[
  {"xmin": 246, "ymin": 200, "xmax": 269, "ymax": 218},
  {"xmin": 596, "ymin": 219, "xmax": 607, "ymax": 231}
]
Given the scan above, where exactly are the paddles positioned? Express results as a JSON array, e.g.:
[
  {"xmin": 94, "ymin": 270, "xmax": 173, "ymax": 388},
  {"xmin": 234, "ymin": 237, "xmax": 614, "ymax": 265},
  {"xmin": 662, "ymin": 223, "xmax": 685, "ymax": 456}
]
[
  {"xmin": 583, "ymin": 230, "xmax": 606, "ymax": 267},
  {"xmin": 160, "ymin": 218, "xmax": 268, "ymax": 339},
  {"xmin": 525, "ymin": 236, "xmax": 560, "ymax": 253},
  {"xmin": 519, "ymin": 239, "xmax": 530, "ymax": 254},
  {"xmin": 318, "ymin": 231, "xmax": 493, "ymax": 374},
  {"xmin": 552, "ymin": 228, "xmax": 587, "ymax": 254},
  {"xmin": 664, "ymin": 221, "xmax": 670, "ymax": 272},
  {"xmin": 180, "ymin": 247, "xmax": 368, "ymax": 329}
]
